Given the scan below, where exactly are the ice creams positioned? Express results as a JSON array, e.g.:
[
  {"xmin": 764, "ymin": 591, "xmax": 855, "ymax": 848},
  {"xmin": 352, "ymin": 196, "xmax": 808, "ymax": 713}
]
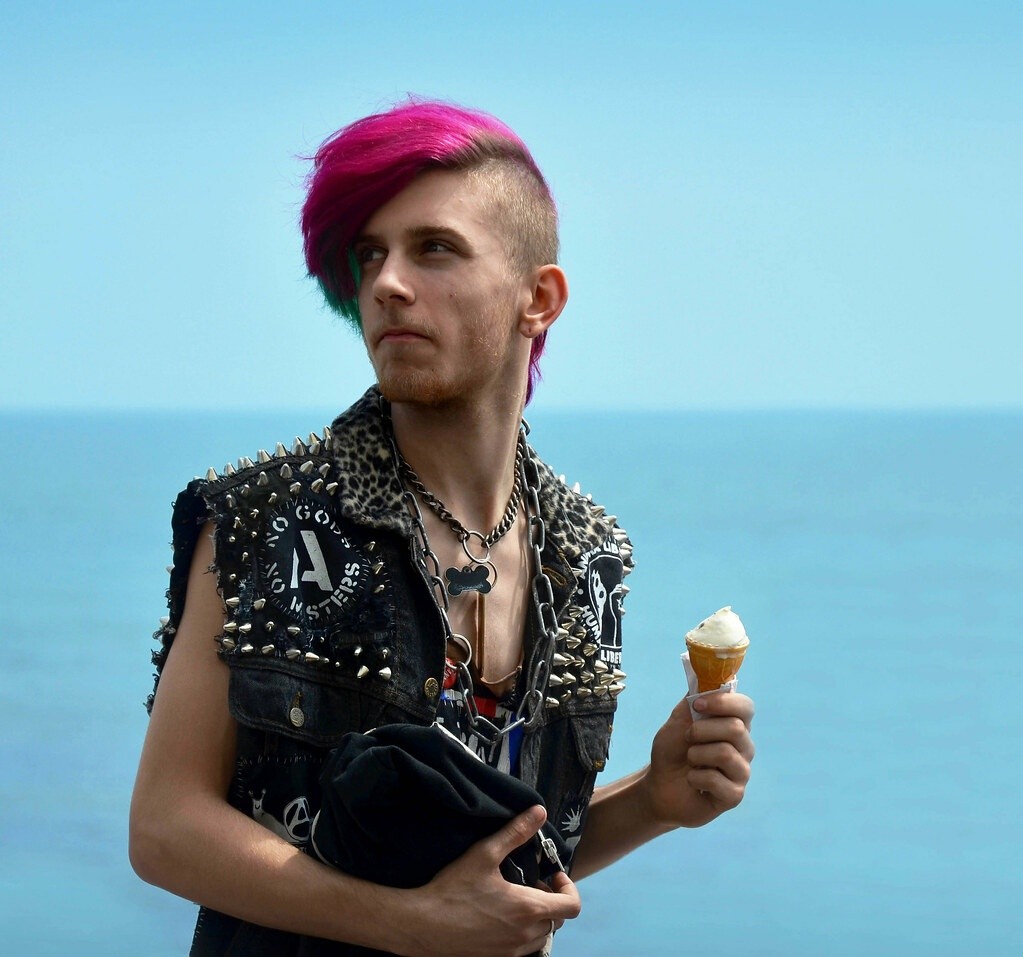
[{"xmin": 686, "ymin": 606, "xmax": 747, "ymax": 691}]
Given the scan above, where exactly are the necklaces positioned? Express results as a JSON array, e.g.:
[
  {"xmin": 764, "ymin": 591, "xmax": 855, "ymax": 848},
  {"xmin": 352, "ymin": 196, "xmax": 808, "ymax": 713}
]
[{"xmin": 376, "ymin": 393, "xmax": 562, "ymax": 747}]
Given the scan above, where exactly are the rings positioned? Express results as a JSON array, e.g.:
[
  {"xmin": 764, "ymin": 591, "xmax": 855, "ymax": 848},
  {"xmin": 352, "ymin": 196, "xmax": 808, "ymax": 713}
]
[{"xmin": 545, "ymin": 917, "xmax": 555, "ymax": 939}]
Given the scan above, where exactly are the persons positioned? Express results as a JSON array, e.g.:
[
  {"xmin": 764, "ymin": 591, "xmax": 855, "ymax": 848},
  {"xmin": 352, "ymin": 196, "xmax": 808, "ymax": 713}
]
[{"xmin": 124, "ymin": 91, "xmax": 758, "ymax": 957}]
